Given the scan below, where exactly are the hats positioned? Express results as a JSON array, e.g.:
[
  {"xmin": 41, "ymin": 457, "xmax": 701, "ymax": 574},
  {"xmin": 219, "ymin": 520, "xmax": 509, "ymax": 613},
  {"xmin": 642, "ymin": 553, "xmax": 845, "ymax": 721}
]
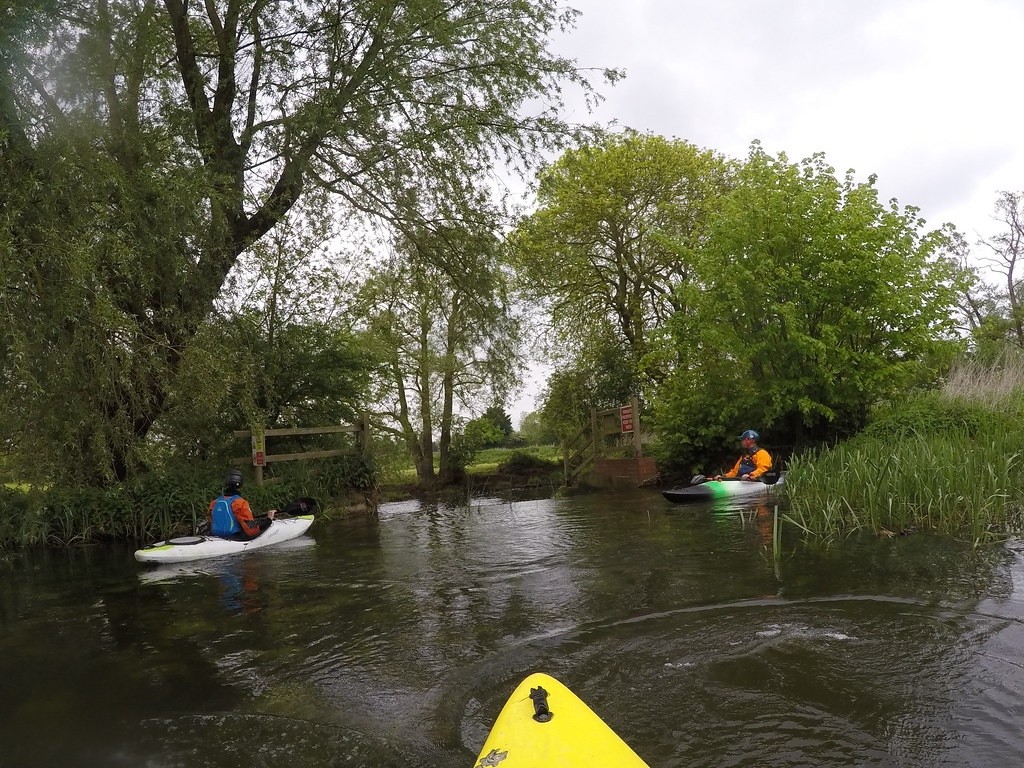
[
  {"xmin": 737, "ymin": 430, "xmax": 760, "ymax": 441},
  {"xmin": 226, "ymin": 469, "xmax": 244, "ymax": 483}
]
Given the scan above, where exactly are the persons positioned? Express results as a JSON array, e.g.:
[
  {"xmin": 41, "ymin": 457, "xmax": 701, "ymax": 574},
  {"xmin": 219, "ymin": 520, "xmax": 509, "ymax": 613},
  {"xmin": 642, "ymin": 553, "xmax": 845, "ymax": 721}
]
[
  {"xmin": 207, "ymin": 468, "xmax": 278, "ymax": 543},
  {"xmin": 712, "ymin": 429, "xmax": 773, "ymax": 482}
]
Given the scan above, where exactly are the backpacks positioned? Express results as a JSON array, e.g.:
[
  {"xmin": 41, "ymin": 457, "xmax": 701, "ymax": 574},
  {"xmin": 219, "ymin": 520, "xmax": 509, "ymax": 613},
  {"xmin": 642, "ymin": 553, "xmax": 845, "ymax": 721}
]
[{"xmin": 212, "ymin": 495, "xmax": 243, "ymax": 536}]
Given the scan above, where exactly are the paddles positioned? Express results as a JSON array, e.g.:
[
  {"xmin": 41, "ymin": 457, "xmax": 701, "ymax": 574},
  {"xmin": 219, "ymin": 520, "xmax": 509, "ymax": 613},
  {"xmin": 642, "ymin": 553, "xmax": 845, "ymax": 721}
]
[
  {"xmin": 194, "ymin": 497, "xmax": 318, "ymax": 536},
  {"xmin": 691, "ymin": 468, "xmax": 781, "ymax": 485}
]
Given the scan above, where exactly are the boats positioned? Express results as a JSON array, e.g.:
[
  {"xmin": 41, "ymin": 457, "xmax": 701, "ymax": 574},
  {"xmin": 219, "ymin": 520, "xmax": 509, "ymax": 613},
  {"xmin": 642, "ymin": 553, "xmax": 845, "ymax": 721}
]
[
  {"xmin": 661, "ymin": 471, "xmax": 790, "ymax": 504},
  {"xmin": 135, "ymin": 513, "xmax": 316, "ymax": 564}
]
